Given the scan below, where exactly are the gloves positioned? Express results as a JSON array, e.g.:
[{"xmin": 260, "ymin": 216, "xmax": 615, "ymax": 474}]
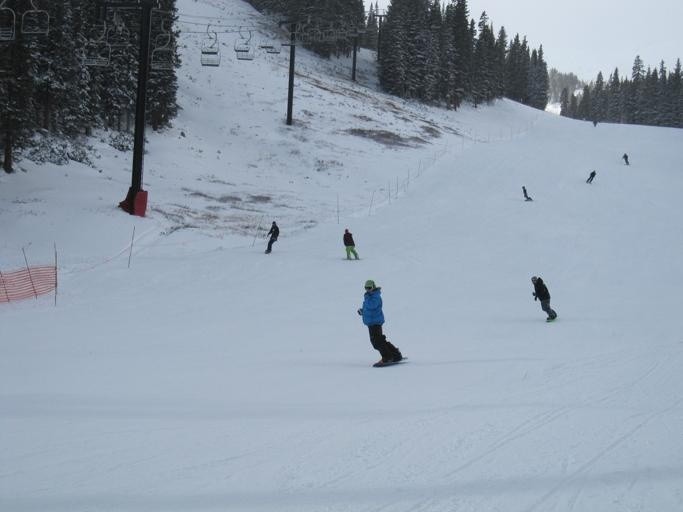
[{"xmin": 358, "ymin": 309, "xmax": 363, "ymax": 315}]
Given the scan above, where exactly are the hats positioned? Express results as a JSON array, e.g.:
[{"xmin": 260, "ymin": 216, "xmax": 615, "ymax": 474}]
[
  {"xmin": 365, "ymin": 281, "xmax": 375, "ymax": 288},
  {"xmin": 531, "ymin": 277, "xmax": 538, "ymax": 280}
]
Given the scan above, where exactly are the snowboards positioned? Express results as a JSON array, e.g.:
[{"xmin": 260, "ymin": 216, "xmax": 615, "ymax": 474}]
[{"xmin": 373, "ymin": 356, "xmax": 407, "ymax": 368}]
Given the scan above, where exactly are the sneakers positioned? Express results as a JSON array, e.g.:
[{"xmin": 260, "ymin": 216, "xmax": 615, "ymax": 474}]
[
  {"xmin": 547, "ymin": 313, "xmax": 556, "ymax": 320},
  {"xmin": 374, "ymin": 354, "xmax": 402, "ymax": 366}
]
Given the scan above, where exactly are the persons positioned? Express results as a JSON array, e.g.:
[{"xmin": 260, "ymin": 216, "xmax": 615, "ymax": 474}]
[
  {"xmin": 341, "ymin": 227, "xmax": 360, "ymax": 261},
  {"xmin": 584, "ymin": 169, "xmax": 596, "ymax": 185},
  {"xmin": 356, "ymin": 278, "xmax": 403, "ymax": 367},
  {"xmin": 621, "ymin": 153, "xmax": 629, "ymax": 165},
  {"xmin": 530, "ymin": 274, "xmax": 557, "ymax": 323},
  {"xmin": 521, "ymin": 185, "xmax": 531, "ymax": 202},
  {"xmin": 262, "ymin": 220, "xmax": 280, "ymax": 254}
]
